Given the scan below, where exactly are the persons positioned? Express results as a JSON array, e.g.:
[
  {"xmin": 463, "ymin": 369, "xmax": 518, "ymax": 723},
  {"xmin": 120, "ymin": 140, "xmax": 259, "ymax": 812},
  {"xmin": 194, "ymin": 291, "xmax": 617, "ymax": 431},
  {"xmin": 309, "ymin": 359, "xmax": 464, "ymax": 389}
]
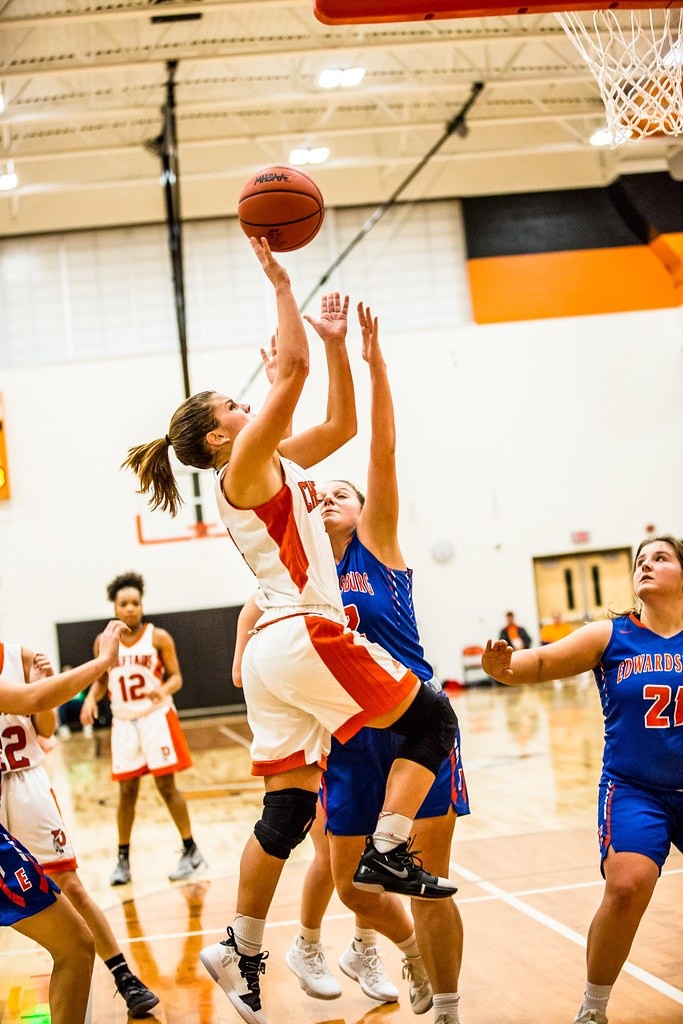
[
  {"xmin": 0, "ymin": 633, "xmax": 160, "ymax": 1019},
  {"xmin": 0, "ymin": 618, "xmax": 130, "ymax": 1019},
  {"xmin": 500, "ymin": 612, "xmax": 531, "ymax": 652},
  {"xmin": 57, "ymin": 664, "xmax": 93, "ymax": 740},
  {"xmin": 541, "ymin": 610, "xmax": 572, "ymax": 645},
  {"xmin": 173, "ymin": 237, "xmax": 460, "ymax": 1017},
  {"xmin": 256, "ymin": 308, "xmax": 464, "ymax": 1024},
  {"xmin": 81, "ymin": 571, "xmax": 203, "ymax": 885},
  {"xmin": 232, "ymin": 589, "xmax": 399, "ymax": 1002},
  {"xmin": 480, "ymin": 538, "xmax": 683, "ymax": 1024}
]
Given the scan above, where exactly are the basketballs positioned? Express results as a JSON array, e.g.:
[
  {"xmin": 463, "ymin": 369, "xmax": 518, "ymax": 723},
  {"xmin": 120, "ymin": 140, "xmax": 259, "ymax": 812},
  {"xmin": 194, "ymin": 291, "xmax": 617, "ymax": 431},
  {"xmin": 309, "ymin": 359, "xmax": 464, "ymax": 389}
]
[{"xmin": 236, "ymin": 165, "xmax": 323, "ymax": 252}]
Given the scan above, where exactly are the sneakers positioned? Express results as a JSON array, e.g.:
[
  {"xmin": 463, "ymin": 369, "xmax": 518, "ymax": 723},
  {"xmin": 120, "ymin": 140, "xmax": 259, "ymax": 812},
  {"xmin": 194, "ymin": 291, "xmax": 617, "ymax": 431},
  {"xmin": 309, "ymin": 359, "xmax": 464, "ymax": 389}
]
[
  {"xmin": 168, "ymin": 844, "xmax": 203, "ymax": 882},
  {"xmin": 573, "ymin": 1001, "xmax": 606, "ymax": 1024},
  {"xmin": 110, "ymin": 860, "xmax": 130, "ymax": 884},
  {"xmin": 283, "ymin": 940, "xmax": 400, "ymax": 1001},
  {"xmin": 199, "ymin": 925, "xmax": 270, "ymax": 1024},
  {"xmin": 353, "ymin": 836, "xmax": 457, "ymax": 901},
  {"xmin": 113, "ymin": 972, "xmax": 159, "ymax": 1016},
  {"xmin": 404, "ymin": 952, "xmax": 459, "ymax": 1023}
]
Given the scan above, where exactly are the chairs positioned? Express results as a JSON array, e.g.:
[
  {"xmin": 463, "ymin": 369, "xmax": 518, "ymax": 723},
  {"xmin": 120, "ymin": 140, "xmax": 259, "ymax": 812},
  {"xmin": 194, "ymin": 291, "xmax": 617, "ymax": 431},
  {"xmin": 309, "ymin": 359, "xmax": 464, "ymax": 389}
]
[{"xmin": 462, "ymin": 644, "xmax": 497, "ymax": 688}]
